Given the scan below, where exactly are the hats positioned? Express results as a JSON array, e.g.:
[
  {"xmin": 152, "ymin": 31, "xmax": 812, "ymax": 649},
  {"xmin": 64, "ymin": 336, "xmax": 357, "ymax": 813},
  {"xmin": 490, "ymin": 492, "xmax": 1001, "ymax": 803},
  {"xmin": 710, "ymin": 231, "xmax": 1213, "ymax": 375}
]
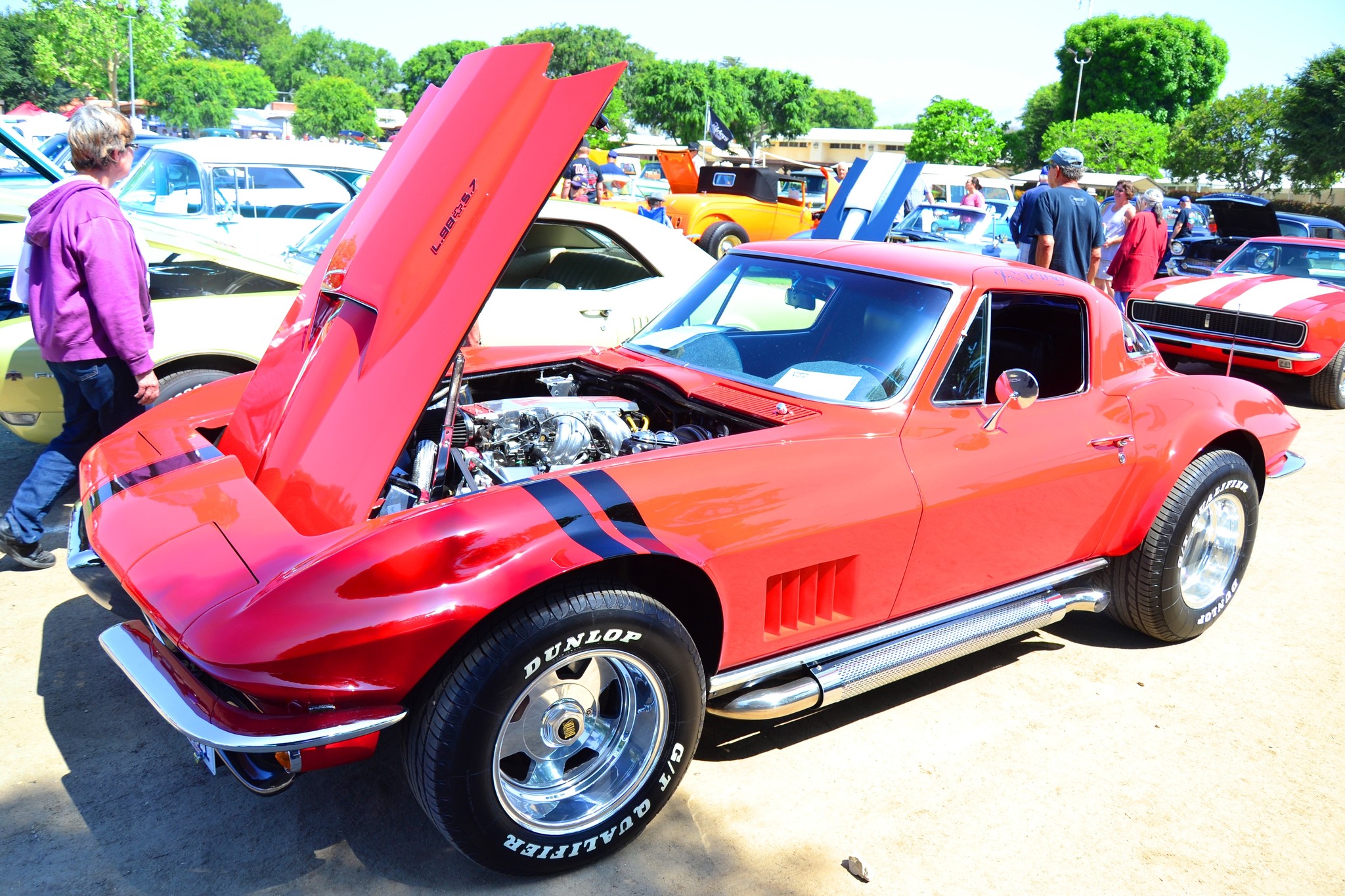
[
  {"xmin": 1143, "ymin": 188, "xmax": 1164, "ymax": 203},
  {"xmin": 647, "ymin": 192, "xmax": 666, "ymax": 201},
  {"xmin": 1177, "ymin": 196, "xmax": 1190, "ymax": 205},
  {"xmin": 581, "ymin": 138, "xmax": 590, "ymax": 148},
  {"xmin": 1043, "ymin": 147, "xmax": 1083, "ymax": 168},
  {"xmin": 1040, "ymin": 165, "xmax": 1050, "ymax": 175},
  {"xmin": 567, "ymin": 174, "xmax": 588, "ymax": 188},
  {"xmin": 1086, "ymin": 187, "xmax": 1098, "ymax": 196},
  {"xmin": 608, "ymin": 150, "xmax": 619, "ymax": 159}
]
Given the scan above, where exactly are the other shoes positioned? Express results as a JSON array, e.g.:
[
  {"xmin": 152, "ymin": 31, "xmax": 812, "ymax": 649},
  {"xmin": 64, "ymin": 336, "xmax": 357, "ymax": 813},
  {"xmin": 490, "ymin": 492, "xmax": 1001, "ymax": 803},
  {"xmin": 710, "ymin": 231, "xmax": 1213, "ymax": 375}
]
[{"xmin": 0, "ymin": 516, "xmax": 56, "ymax": 569}]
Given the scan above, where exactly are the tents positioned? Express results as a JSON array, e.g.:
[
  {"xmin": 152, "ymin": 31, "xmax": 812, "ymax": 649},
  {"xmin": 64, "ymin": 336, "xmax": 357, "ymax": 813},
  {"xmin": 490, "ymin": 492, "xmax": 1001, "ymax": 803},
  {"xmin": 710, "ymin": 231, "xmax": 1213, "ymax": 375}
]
[
  {"xmin": 61, "ymin": 103, "xmax": 84, "ymax": 119},
  {"xmin": 228, "ymin": 109, "xmax": 284, "ymax": 133},
  {"xmin": 7, "ymin": 100, "xmax": 48, "ymax": 116}
]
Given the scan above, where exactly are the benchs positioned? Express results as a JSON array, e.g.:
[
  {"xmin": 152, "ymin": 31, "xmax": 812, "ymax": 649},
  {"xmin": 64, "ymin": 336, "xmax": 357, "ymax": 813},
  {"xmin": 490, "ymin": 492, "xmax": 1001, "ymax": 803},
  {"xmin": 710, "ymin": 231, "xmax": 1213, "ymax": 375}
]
[{"xmin": 540, "ymin": 250, "xmax": 653, "ymax": 289}]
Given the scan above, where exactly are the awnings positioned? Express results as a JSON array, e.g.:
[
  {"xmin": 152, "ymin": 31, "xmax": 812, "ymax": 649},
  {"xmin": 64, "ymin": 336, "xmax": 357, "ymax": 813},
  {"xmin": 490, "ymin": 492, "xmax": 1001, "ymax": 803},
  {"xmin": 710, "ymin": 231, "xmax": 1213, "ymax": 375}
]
[
  {"xmin": 611, "ymin": 145, "xmax": 725, "ymax": 163},
  {"xmin": 1008, "ymin": 169, "xmax": 1169, "ymax": 197},
  {"xmin": 920, "ymin": 163, "xmax": 1011, "ymax": 181}
]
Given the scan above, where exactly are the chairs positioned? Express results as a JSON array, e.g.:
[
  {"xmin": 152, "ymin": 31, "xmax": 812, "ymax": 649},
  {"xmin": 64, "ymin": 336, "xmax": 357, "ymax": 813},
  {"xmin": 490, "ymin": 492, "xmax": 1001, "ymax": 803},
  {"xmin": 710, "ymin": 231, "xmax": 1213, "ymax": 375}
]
[
  {"xmin": 863, "ymin": 297, "xmax": 916, "ymax": 337},
  {"xmin": 1273, "ymin": 266, "xmax": 1310, "ymax": 278},
  {"xmin": 975, "ymin": 325, "xmax": 1052, "ymax": 398}
]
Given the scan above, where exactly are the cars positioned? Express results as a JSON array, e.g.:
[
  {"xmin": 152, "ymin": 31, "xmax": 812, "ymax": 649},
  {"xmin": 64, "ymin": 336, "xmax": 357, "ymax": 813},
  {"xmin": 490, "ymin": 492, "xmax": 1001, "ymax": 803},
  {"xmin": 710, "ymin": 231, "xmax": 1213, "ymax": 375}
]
[
  {"xmin": 81, "ymin": 43, "xmax": 1307, "ymax": 865},
  {"xmin": 0, "ymin": 94, "xmax": 1057, "ymax": 299},
  {"xmin": 117, "ymin": 194, "xmax": 826, "ymax": 412},
  {"xmin": 1128, "ymin": 235, "xmax": 1345, "ymax": 410},
  {"xmin": 1096, "ymin": 196, "xmax": 1215, "ymax": 273},
  {"xmin": 1166, "ymin": 194, "xmax": 1345, "ymax": 276}
]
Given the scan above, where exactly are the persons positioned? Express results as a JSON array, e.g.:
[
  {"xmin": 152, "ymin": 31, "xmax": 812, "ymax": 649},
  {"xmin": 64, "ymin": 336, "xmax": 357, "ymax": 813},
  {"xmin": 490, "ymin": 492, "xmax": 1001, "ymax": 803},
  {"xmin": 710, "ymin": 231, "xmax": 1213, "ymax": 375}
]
[
  {"xmin": 567, "ymin": 175, "xmax": 589, "ymax": 203},
  {"xmin": 1086, "ymin": 187, "xmax": 1100, "ymax": 207},
  {"xmin": 0, "ymin": 104, "xmax": 160, "ymax": 570},
  {"xmin": 161, "ymin": 125, "xmax": 190, "ymax": 139},
  {"xmin": 946, "ymin": 176, "xmax": 985, "ymax": 232},
  {"xmin": 686, "ymin": 142, "xmax": 706, "ymax": 176},
  {"xmin": 599, "ymin": 151, "xmax": 625, "ymax": 174},
  {"xmin": 1105, "ymin": 188, "xmax": 1168, "ymax": 313},
  {"xmin": 904, "ymin": 159, "xmax": 942, "ymax": 241},
  {"xmin": 1027, "ymin": 147, "xmax": 1107, "ymax": 284},
  {"xmin": 1009, "ymin": 166, "xmax": 1053, "ymax": 266},
  {"xmin": 561, "ymin": 139, "xmax": 604, "ymax": 205},
  {"xmin": 245, "ymin": 132, "xmax": 377, "ymax": 147},
  {"xmin": 1168, "ymin": 196, "xmax": 1196, "ymax": 250},
  {"xmin": 1094, "ymin": 179, "xmax": 1143, "ymax": 299},
  {"xmin": 824, "ymin": 161, "xmax": 848, "ymax": 206},
  {"xmin": 647, "ymin": 191, "xmax": 674, "ymax": 229},
  {"xmin": 892, "ymin": 202, "xmax": 906, "ymax": 244}
]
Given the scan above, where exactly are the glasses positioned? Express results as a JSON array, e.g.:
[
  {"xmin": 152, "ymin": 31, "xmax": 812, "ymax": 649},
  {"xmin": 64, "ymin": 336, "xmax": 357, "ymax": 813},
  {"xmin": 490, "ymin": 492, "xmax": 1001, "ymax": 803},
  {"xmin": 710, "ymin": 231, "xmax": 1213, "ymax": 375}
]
[
  {"xmin": 967, "ymin": 181, "xmax": 970, "ymax": 183},
  {"xmin": 1114, "ymin": 186, "xmax": 1126, "ymax": 192},
  {"xmin": 570, "ymin": 184, "xmax": 582, "ymax": 190},
  {"xmin": 125, "ymin": 142, "xmax": 139, "ymax": 152}
]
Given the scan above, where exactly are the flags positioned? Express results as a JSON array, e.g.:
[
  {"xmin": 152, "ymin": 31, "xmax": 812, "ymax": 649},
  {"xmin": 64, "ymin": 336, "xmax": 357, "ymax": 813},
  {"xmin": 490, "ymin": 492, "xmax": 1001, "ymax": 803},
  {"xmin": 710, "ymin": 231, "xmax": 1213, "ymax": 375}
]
[{"xmin": 707, "ymin": 105, "xmax": 734, "ymax": 150}]
